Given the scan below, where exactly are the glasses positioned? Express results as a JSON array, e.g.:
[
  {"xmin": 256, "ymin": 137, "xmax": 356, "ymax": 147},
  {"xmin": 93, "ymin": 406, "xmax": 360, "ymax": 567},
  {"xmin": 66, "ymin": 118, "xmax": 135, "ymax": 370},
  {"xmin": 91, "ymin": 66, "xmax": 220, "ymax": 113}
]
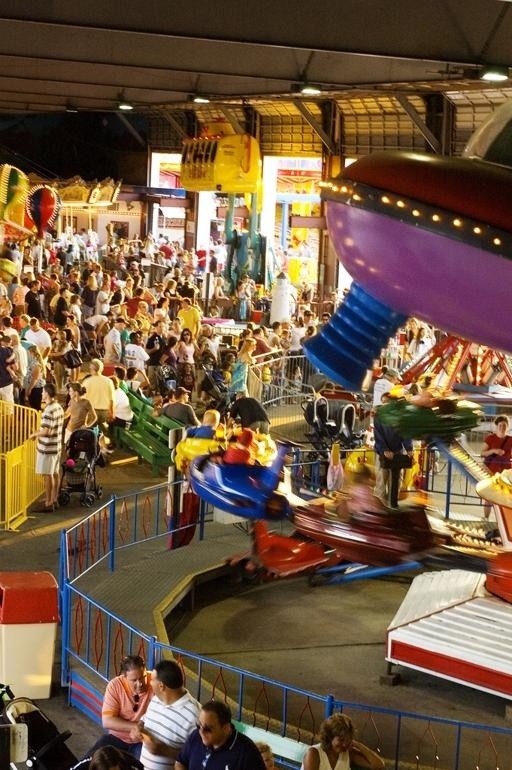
[
  {"xmin": 195, "ymin": 721, "xmax": 212, "ymax": 733},
  {"xmin": 133, "ymin": 694, "xmax": 140, "ymax": 712}
]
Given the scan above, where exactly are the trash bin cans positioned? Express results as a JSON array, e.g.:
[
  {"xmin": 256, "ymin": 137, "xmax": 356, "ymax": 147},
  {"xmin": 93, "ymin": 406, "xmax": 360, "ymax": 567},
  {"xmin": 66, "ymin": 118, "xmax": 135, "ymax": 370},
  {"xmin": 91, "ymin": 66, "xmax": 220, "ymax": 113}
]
[{"xmin": 0, "ymin": 570, "xmax": 61, "ymax": 702}]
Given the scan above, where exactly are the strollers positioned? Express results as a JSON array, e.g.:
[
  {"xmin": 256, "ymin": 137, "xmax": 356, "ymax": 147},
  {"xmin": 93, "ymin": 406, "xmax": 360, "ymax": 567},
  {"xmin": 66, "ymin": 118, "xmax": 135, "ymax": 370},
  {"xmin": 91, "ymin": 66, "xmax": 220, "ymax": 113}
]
[
  {"xmin": 0, "ymin": 682, "xmax": 88, "ymax": 770},
  {"xmin": 56, "ymin": 429, "xmax": 104, "ymax": 508},
  {"xmin": 300, "ymin": 397, "xmax": 366, "ymax": 462}
]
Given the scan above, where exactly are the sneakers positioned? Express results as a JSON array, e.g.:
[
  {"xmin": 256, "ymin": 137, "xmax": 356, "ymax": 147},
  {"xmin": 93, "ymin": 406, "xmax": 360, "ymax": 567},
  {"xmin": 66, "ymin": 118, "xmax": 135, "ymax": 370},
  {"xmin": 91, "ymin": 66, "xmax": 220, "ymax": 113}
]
[{"xmin": 31, "ymin": 498, "xmax": 59, "ymax": 512}]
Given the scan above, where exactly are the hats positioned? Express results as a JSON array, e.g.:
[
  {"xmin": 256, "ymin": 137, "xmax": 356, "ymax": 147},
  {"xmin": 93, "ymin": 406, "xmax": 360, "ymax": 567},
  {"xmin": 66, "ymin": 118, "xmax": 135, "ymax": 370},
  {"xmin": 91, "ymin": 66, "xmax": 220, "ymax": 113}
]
[
  {"xmin": 114, "ymin": 319, "xmax": 129, "ymax": 325},
  {"xmin": 174, "ymin": 387, "xmax": 191, "ymax": 398}
]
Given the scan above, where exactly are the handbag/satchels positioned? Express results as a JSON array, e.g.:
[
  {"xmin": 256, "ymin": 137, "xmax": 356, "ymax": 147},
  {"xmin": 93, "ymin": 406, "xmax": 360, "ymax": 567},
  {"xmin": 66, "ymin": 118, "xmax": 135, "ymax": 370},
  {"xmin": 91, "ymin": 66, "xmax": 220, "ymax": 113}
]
[
  {"xmin": 63, "ymin": 344, "xmax": 83, "ymax": 368},
  {"xmin": 483, "ymin": 453, "xmax": 494, "ymax": 465}
]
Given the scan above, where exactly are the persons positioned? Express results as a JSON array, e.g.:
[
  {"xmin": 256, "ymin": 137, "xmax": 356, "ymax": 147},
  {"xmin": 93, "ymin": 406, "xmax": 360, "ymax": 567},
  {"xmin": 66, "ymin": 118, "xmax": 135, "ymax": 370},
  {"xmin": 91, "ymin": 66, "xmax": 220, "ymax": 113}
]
[
  {"xmin": 82, "ymin": 656, "xmax": 367, "ymax": 770},
  {"xmin": 1, "ymin": 225, "xmax": 350, "ymax": 512},
  {"xmin": 372, "ymin": 392, "xmax": 414, "ymax": 510},
  {"xmin": 480, "ymin": 415, "xmax": 512, "ymax": 522},
  {"xmin": 381, "ymin": 316, "xmax": 438, "ymax": 364}
]
